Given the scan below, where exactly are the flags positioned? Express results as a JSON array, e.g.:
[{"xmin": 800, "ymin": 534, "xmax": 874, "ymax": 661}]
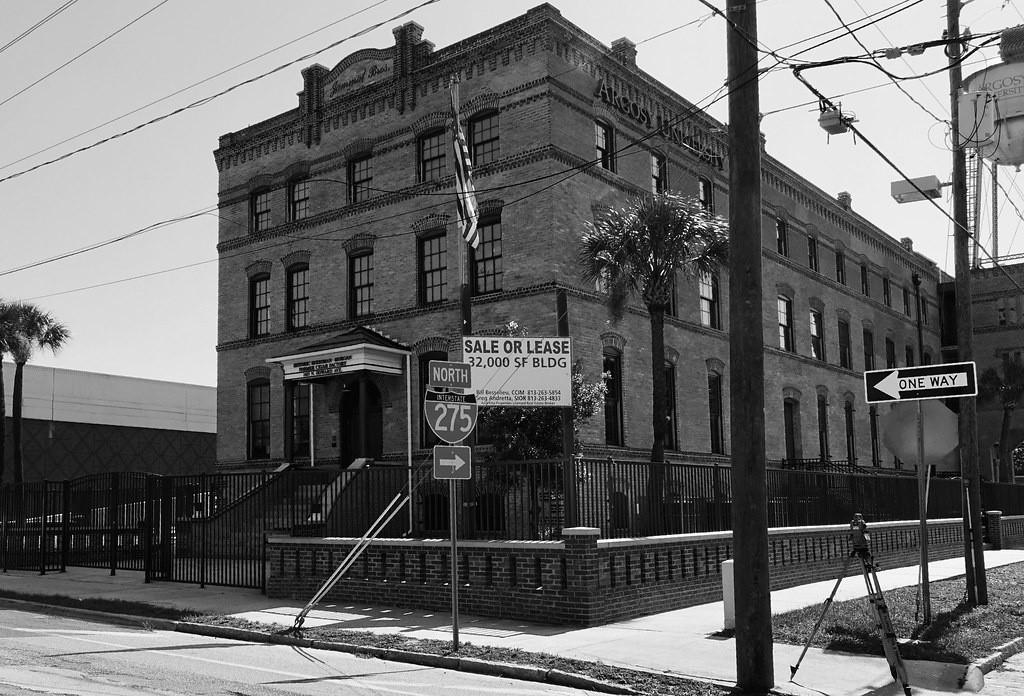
[{"xmin": 448, "ymin": 75, "xmax": 480, "ymax": 250}]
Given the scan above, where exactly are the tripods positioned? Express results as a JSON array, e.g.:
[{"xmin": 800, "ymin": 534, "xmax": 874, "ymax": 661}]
[{"xmin": 790, "ymin": 513, "xmax": 911, "ymax": 696}]
